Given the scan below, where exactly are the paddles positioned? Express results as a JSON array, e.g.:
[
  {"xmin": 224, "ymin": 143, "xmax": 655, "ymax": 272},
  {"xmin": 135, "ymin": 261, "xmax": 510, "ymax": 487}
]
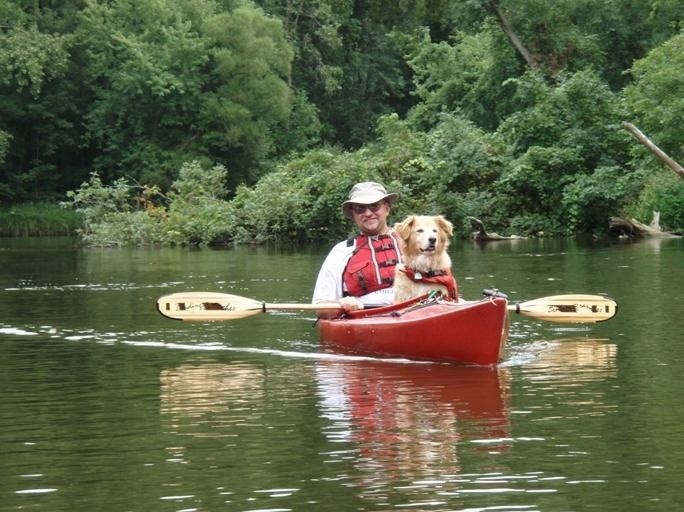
[
  {"xmin": 507, "ymin": 293, "xmax": 618, "ymax": 324},
  {"xmin": 156, "ymin": 291, "xmax": 391, "ymax": 322}
]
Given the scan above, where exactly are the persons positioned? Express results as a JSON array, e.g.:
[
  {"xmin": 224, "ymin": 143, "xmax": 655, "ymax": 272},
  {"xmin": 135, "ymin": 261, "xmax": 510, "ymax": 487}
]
[{"xmin": 312, "ymin": 182, "xmax": 405, "ymax": 320}]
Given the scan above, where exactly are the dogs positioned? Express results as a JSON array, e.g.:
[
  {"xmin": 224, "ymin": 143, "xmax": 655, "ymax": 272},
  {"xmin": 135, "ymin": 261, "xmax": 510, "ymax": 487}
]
[{"xmin": 391, "ymin": 215, "xmax": 458, "ymax": 305}]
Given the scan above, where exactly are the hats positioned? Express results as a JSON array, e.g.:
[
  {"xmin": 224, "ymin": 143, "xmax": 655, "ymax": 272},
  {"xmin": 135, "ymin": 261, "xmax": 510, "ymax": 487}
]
[{"xmin": 341, "ymin": 181, "xmax": 400, "ymax": 219}]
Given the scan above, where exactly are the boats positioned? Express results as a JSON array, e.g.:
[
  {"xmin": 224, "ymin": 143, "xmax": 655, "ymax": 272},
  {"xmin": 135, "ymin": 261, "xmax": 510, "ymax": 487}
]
[{"xmin": 317, "ymin": 297, "xmax": 510, "ymax": 365}]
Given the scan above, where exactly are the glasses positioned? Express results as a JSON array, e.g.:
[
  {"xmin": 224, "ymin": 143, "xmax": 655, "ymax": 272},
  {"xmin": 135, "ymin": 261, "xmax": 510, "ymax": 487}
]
[{"xmin": 351, "ymin": 202, "xmax": 381, "ymax": 214}]
